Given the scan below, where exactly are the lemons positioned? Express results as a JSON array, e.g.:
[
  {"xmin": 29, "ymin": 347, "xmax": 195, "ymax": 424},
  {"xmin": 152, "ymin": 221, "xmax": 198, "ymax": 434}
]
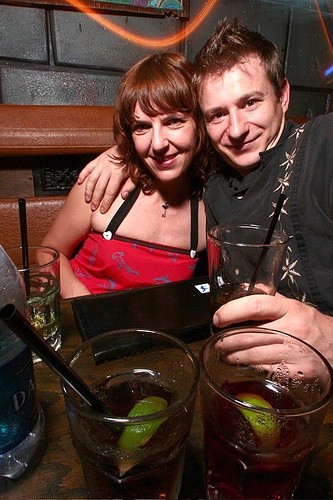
[
  {"xmin": 116, "ymin": 395, "xmax": 167, "ymax": 477},
  {"xmin": 232, "ymin": 392, "xmax": 281, "ymax": 453}
]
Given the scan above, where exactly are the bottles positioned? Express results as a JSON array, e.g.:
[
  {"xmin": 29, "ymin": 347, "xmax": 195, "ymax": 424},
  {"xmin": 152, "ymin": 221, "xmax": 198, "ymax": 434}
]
[{"xmin": 0, "ymin": 245, "xmax": 49, "ymax": 489}]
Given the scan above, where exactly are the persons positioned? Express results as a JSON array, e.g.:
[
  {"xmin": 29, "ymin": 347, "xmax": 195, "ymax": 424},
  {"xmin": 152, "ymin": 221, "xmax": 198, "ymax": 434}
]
[
  {"xmin": 35, "ymin": 50, "xmax": 212, "ymax": 299},
  {"xmin": 78, "ymin": 16, "xmax": 333, "ymax": 382}
]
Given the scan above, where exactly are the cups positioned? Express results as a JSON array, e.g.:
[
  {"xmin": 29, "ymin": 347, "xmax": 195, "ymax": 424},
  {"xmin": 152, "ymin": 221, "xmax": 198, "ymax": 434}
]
[
  {"xmin": 201, "ymin": 326, "xmax": 333, "ymax": 500},
  {"xmin": 206, "ymin": 224, "xmax": 289, "ymax": 337},
  {"xmin": 60, "ymin": 329, "xmax": 200, "ymax": 499},
  {"xmin": 3, "ymin": 245, "xmax": 62, "ymax": 364}
]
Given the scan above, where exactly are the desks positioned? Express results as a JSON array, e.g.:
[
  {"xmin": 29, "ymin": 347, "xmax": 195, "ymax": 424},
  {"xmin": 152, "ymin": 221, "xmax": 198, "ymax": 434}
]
[{"xmin": 0, "ymin": 281, "xmax": 333, "ymax": 500}]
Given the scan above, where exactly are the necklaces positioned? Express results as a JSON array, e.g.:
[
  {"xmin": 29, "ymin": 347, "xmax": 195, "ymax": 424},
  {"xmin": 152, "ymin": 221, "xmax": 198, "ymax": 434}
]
[{"xmin": 161, "ymin": 185, "xmax": 171, "ymax": 218}]
